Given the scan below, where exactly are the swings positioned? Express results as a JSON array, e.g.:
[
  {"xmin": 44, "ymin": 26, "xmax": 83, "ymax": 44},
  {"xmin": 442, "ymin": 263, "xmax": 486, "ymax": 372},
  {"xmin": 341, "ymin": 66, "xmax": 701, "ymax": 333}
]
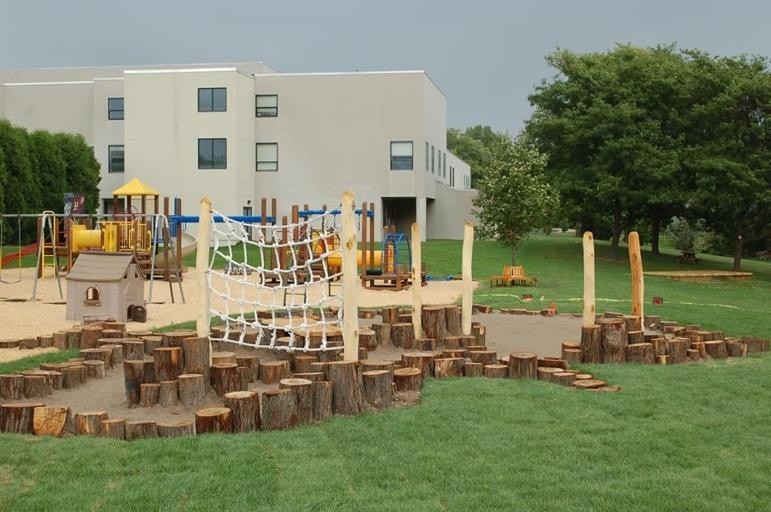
[{"xmin": 1, "ymin": 217, "xmax": 21, "ymax": 284}]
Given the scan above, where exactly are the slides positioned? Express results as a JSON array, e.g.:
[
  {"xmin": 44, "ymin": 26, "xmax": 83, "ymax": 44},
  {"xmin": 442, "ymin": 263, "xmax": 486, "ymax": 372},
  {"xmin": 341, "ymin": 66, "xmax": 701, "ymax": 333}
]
[{"xmin": 150, "ymin": 231, "xmax": 197, "ymax": 268}]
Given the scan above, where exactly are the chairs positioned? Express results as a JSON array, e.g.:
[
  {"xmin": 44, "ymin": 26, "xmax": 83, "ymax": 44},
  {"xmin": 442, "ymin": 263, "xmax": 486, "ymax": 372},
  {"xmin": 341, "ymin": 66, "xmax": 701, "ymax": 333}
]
[{"xmin": 490, "ymin": 265, "xmax": 537, "ymax": 288}]
